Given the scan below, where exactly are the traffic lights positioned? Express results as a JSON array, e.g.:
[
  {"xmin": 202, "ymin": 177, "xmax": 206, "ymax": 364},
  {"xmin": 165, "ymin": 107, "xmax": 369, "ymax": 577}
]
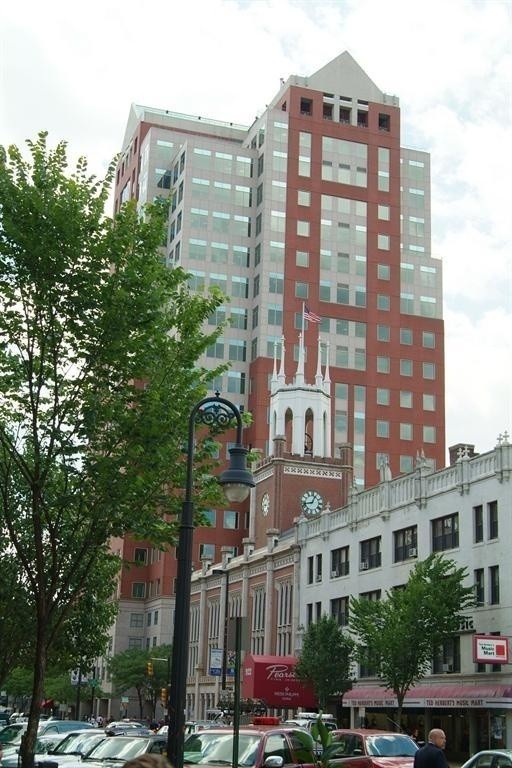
[
  {"xmin": 160, "ymin": 688, "xmax": 168, "ymax": 701},
  {"xmin": 147, "ymin": 662, "xmax": 153, "ymax": 676}
]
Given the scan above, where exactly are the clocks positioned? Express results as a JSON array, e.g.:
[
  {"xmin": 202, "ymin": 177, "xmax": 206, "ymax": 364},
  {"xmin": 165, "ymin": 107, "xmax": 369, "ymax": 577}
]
[{"xmin": 299, "ymin": 490, "xmax": 323, "ymax": 517}]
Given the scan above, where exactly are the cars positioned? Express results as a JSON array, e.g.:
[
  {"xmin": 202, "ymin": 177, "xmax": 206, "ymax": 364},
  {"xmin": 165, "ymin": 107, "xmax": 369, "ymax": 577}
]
[
  {"xmin": 458, "ymin": 749, "xmax": 512, "ymax": 768},
  {"xmin": 0, "ymin": 704, "xmax": 338, "ymax": 768},
  {"xmin": 314, "ymin": 729, "xmax": 423, "ymax": 768}
]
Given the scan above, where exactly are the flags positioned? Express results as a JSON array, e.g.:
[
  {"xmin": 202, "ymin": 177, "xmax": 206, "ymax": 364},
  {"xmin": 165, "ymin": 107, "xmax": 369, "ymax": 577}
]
[{"xmin": 304, "ymin": 303, "xmax": 321, "ymax": 324}]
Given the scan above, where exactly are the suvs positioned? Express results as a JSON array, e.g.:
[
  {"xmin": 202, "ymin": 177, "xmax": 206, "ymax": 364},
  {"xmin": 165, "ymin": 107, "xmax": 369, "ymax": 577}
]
[{"xmin": 181, "ymin": 716, "xmax": 320, "ymax": 768}]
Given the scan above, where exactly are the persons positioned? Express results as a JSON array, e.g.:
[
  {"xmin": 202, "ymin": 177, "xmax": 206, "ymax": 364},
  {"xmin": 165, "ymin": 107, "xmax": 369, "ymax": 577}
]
[
  {"xmin": 82, "ymin": 713, "xmax": 165, "ymax": 731},
  {"xmin": 410, "ymin": 726, "xmax": 421, "ymax": 742},
  {"xmin": 414, "ymin": 728, "xmax": 448, "ymax": 768}
]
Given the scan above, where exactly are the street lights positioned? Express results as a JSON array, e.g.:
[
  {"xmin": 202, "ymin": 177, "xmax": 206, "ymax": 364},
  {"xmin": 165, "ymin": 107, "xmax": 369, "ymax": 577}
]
[
  {"xmin": 211, "ymin": 568, "xmax": 230, "ymax": 712},
  {"xmin": 168, "ymin": 390, "xmax": 256, "ymax": 768}
]
